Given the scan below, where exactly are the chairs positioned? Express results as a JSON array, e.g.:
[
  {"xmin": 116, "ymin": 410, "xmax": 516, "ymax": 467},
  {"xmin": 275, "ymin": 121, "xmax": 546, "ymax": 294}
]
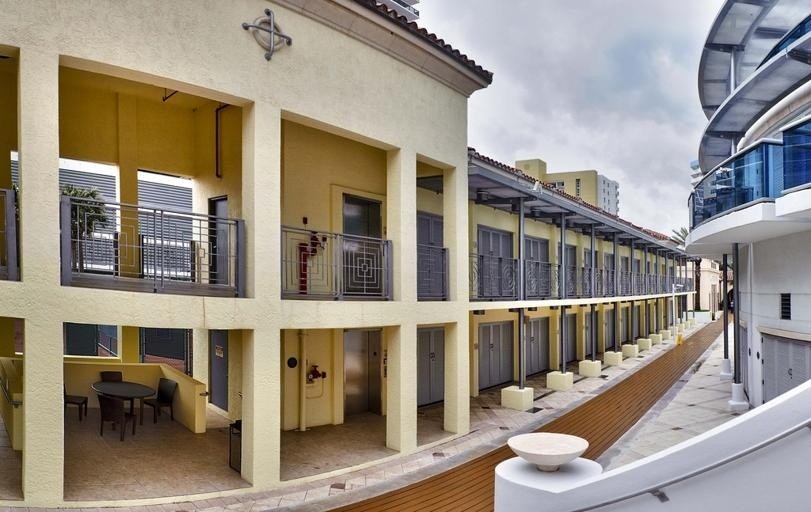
[
  {"xmin": 64, "ymin": 383, "xmax": 88, "ymax": 422},
  {"xmin": 143, "ymin": 377, "xmax": 178, "ymax": 425},
  {"xmin": 95, "ymin": 393, "xmax": 138, "ymax": 442},
  {"xmin": 100, "ymin": 371, "xmax": 124, "ymax": 410}
]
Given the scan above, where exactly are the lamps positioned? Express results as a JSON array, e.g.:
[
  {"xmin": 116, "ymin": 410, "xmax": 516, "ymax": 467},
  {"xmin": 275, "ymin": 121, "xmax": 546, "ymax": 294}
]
[{"xmin": 242, "ymin": 8, "xmax": 293, "ymax": 63}]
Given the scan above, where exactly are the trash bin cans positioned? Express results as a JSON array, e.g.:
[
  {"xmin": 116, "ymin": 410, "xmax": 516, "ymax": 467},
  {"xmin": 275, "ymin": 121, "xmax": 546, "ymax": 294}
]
[{"xmin": 229, "ymin": 420, "xmax": 241, "ymax": 474}]
